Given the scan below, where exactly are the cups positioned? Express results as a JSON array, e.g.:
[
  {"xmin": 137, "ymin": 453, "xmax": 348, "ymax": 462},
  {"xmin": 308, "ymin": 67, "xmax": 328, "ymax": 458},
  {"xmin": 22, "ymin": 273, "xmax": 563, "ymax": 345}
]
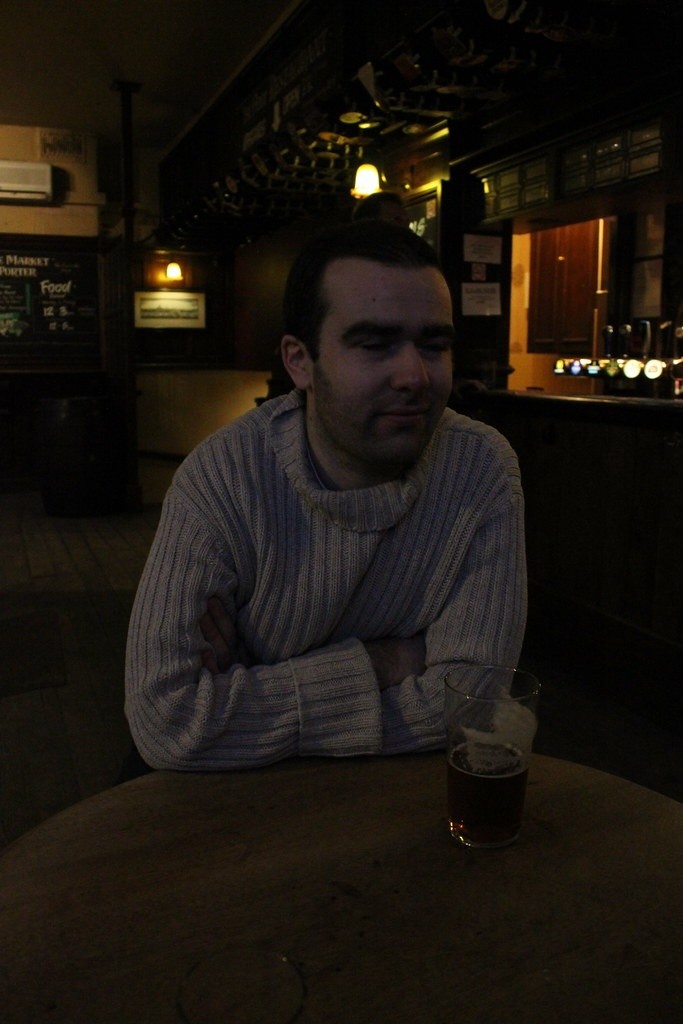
[{"xmin": 443, "ymin": 665, "xmax": 543, "ymax": 850}]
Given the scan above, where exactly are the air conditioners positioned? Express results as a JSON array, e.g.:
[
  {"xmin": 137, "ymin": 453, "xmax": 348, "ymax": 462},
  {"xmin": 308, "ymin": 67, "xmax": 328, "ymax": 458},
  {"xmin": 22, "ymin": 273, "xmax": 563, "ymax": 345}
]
[{"xmin": 0, "ymin": 160, "xmax": 52, "ymax": 207}]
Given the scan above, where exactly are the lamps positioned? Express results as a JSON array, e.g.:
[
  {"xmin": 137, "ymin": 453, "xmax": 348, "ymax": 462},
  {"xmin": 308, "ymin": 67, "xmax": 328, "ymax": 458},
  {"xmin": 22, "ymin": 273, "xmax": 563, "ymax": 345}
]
[{"xmin": 348, "ymin": 164, "xmax": 385, "ymax": 202}]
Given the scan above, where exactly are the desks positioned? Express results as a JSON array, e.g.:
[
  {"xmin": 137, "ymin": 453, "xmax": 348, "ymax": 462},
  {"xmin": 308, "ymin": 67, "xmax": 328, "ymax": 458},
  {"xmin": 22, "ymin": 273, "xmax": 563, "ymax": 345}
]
[{"xmin": 0, "ymin": 744, "xmax": 683, "ymax": 1024}]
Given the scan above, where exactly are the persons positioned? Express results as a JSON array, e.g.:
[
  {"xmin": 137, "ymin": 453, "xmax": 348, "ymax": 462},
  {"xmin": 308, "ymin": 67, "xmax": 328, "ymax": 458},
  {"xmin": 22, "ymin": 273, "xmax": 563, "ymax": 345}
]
[{"xmin": 122, "ymin": 217, "xmax": 529, "ymax": 771}]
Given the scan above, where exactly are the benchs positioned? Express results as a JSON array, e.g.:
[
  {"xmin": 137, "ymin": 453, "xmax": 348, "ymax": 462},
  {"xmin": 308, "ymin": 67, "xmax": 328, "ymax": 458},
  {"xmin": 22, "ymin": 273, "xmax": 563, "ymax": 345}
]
[{"xmin": 446, "ymin": 393, "xmax": 683, "ymax": 735}]
